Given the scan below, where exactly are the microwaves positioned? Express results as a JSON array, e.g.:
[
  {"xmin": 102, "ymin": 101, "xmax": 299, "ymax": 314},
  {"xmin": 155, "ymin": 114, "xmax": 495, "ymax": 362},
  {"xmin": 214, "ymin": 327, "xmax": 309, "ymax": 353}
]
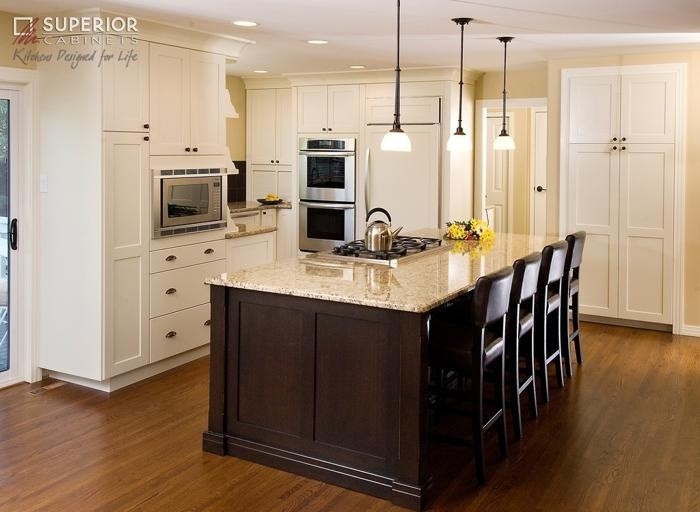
[{"xmin": 161, "ymin": 177, "xmax": 222, "ymax": 228}]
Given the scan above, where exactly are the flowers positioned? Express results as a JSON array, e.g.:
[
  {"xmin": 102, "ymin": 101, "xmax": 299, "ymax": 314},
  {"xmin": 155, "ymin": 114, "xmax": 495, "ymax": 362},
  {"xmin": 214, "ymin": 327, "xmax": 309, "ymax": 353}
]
[
  {"xmin": 451, "ymin": 241, "xmax": 497, "ymax": 261},
  {"xmin": 443, "ymin": 219, "xmax": 495, "ymax": 241}
]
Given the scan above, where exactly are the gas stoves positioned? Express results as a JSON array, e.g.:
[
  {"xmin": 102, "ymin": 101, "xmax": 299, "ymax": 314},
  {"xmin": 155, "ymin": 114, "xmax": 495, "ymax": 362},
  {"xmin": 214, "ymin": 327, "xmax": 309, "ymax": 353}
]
[{"xmin": 315, "ymin": 235, "xmax": 453, "ymax": 263}]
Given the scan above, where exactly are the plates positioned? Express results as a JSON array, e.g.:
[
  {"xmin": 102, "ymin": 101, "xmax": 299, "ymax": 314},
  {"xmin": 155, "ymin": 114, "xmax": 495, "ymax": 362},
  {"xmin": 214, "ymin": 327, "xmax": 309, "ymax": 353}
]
[{"xmin": 257, "ymin": 198, "xmax": 283, "ymax": 205}]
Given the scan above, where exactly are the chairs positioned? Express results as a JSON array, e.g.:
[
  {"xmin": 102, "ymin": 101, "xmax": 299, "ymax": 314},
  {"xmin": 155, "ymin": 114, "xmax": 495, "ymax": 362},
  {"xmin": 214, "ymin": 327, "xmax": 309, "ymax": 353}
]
[{"xmin": 428, "ymin": 230, "xmax": 587, "ymax": 484}]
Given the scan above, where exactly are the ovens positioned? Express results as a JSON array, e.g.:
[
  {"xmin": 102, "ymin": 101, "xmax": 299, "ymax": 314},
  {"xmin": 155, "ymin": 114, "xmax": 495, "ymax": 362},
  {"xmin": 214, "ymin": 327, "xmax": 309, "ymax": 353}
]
[{"xmin": 297, "ymin": 137, "xmax": 357, "ymax": 253}]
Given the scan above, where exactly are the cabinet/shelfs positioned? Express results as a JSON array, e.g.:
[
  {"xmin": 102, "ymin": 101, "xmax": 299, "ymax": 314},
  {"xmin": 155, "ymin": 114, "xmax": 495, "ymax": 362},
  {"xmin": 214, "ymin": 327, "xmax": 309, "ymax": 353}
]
[
  {"xmin": 261, "ymin": 209, "xmax": 276, "ymax": 227},
  {"xmin": 40, "ymin": 12, "xmax": 148, "ymax": 393},
  {"xmin": 289, "ymin": 72, "xmax": 363, "ymax": 137},
  {"xmin": 566, "ymin": 144, "xmax": 675, "ymax": 326},
  {"xmin": 147, "ymin": 228, "xmax": 227, "ymax": 383},
  {"xmin": 241, "ymin": 75, "xmax": 296, "ymax": 168},
  {"xmin": 143, "ymin": 20, "xmax": 257, "ymax": 164},
  {"xmin": 246, "ymin": 166, "xmax": 297, "ymax": 266},
  {"xmin": 566, "ymin": 73, "xmax": 675, "ymax": 144}
]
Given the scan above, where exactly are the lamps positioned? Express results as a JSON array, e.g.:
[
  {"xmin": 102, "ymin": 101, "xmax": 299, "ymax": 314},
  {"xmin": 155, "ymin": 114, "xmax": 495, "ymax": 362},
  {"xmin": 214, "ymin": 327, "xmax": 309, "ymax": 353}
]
[
  {"xmin": 381, "ymin": 0, "xmax": 412, "ymax": 154},
  {"xmin": 445, "ymin": 16, "xmax": 476, "ymax": 152},
  {"xmin": 494, "ymin": 36, "xmax": 517, "ymax": 150}
]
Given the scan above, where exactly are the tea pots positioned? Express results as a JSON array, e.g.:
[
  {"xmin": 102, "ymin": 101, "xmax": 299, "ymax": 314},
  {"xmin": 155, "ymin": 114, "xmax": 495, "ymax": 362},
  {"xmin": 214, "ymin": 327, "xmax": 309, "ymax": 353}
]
[{"xmin": 364, "ymin": 207, "xmax": 403, "ymax": 253}]
